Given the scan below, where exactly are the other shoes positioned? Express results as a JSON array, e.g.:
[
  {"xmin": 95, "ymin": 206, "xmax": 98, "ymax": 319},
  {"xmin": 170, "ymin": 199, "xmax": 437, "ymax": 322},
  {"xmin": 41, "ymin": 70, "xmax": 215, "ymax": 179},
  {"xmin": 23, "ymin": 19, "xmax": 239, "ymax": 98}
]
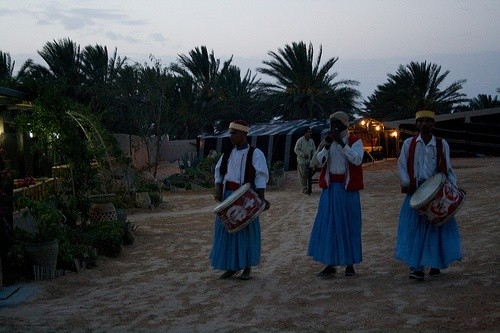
[
  {"xmin": 345, "ymin": 267, "xmax": 354, "ymax": 274},
  {"xmin": 408, "ymin": 271, "xmax": 425, "ymax": 279},
  {"xmin": 429, "ymin": 268, "xmax": 440, "ymax": 276},
  {"xmin": 241, "ymin": 268, "xmax": 251, "ymax": 279},
  {"xmin": 222, "ymin": 270, "xmax": 237, "ymax": 278},
  {"xmin": 318, "ymin": 267, "xmax": 336, "ymax": 276}
]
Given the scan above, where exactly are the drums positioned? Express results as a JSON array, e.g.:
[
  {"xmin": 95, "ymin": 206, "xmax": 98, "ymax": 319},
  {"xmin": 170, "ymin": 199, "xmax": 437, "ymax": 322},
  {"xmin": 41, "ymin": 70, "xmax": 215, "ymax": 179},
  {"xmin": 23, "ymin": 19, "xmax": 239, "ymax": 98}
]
[
  {"xmin": 212, "ymin": 183, "xmax": 266, "ymax": 233},
  {"xmin": 409, "ymin": 172, "xmax": 465, "ymax": 228}
]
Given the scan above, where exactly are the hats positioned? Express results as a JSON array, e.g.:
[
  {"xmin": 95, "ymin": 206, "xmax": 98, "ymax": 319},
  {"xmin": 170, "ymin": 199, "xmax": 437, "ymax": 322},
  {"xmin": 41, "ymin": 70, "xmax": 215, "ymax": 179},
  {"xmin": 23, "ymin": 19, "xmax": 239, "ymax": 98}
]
[
  {"xmin": 330, "ymin": 111, "xmax": 349, "ymax": 127},
  {"xmin": 229, "ymin": 120, "xmax": 250, "ymax": 133},
  {"xmin": 415, "ymin": 110, "xmax": 435, "ymax": 119}
]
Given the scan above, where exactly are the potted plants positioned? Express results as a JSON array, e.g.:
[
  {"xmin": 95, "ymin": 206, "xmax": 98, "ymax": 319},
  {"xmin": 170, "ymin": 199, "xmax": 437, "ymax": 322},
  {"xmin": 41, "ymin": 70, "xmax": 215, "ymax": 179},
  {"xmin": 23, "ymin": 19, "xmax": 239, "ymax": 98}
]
[
  {"xmin": 273, "ymin": 161, "xmax": 284, "ymax": 177},
  {"xmin": 16, "ymin": 195, "xmax": 66, "ymax": 281},
  {"xmin": 88, "ymin": 172, "xmax": 119, "ymax": 222},
  {"xmin": 134, "ymin": 183, "xmax": 150, "ymax": 207}
]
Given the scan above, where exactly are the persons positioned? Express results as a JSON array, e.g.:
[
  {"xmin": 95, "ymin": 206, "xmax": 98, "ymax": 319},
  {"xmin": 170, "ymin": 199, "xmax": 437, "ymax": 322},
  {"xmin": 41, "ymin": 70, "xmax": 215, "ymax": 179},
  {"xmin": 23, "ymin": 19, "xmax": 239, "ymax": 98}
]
[
  {"xmin": 294, "ymin": 127, "xmax": 318, "ymax": 195},
  {"xmin": 307, "ymin": 111, "xmax": 364, "ymax": 278},
  {"xmin": 209, "ymin": 120, "xmax": 270, "ymax": 279},
  {"xmin": 395, "ymin": 110, "xmax": 467, "ymax": 281}
]
